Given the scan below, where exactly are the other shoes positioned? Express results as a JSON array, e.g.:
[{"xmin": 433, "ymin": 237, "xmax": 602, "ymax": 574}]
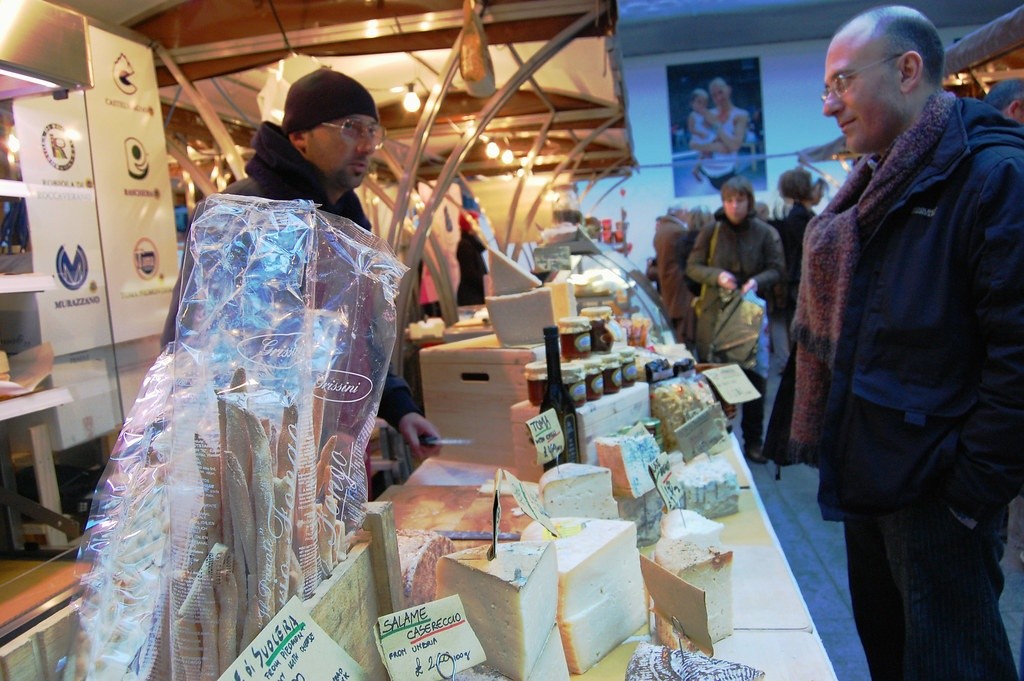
[{"xmin": 743, "ymin": 446, "xmax": 769, "ymax": 464}]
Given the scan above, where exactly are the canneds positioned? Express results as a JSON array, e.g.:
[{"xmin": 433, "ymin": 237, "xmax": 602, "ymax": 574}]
[{"xmin": 523, "ymin": 304, "xmax": 638, "ymax": 408}]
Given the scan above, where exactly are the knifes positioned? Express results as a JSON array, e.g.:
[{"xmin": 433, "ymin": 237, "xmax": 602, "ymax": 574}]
[{"xmin": 404, "ymin": 435, "xmax": 472, "ymax": 445}]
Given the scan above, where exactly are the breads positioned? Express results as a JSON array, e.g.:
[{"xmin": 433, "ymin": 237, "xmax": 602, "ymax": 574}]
[{"xmin": 162, "ymin": 368, "xmax": 734, "ymax": 681}]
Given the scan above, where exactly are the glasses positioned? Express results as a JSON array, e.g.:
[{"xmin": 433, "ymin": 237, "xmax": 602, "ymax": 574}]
[
  {"xmin": 821, "ymin": 53, "xmax": 903, "ymax": 102},
  {"xmin": 321, "ymin": 118, "xmax": 387, "ymax": 151}
]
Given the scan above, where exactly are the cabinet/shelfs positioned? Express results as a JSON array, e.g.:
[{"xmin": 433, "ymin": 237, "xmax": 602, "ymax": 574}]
[{"xmin": 0, "ymin": 0, "xmax": 181, "ymax": 451}]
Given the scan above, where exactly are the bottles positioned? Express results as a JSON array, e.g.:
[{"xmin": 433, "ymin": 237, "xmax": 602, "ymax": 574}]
[
  {"xmin": 539, "ymin": 327, "xmax": 582, "ymax": 473},
  {"xmin": 550, "ymin": 183, "xmax": 578, "ymax": 227}
]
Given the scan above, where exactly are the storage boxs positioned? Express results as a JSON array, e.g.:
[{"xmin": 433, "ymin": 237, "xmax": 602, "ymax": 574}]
[
  {"xmin": 694, "ymin": 363, "xmax": 763, "ymax": 421},
  {"xmin": 419, "ymin": 333, "xmax": 546, "ymax": 458},
  {"xmin": 510, "ymin": 381, "xmax": 651, "ymax": 483}
]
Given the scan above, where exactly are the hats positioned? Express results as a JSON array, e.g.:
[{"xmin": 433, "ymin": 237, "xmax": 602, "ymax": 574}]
[
  {"xmin": 281, "ymin": 68, "xmax": 378, "ymax": 134},
  {"xmin": 459, "ymin": 210, "xmax": 479, "ymax": 231}
]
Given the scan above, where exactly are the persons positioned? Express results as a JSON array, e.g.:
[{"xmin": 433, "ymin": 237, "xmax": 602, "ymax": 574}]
[
  {"xmin": 455, "ymin": 209, "xmax": 490, "ymax": 307},
  {"xmin": 762, "ymin": 6, "xmax": 1024, "ymax": 681},
  {"xmin": 686, "ymin": 77, "xmax": 749, "ymax": 191},
  {"xmin": 161, "ymin": 66, "xmax": 442, "ymax": 499},
  {"xmin": 646, "ymin": 166, "xmax": 826, "ymax": 463}
]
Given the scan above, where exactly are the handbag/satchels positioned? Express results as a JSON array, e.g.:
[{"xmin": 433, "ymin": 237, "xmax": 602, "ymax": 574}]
[
  {"xmin": 736, "ymin": 286, "xmax": 769, "ymax": 380},
  {"xmin": 708, "ymin": 294, "xmax": 762, "ymax": 370},
  {"xmin": 691, "ymin": 220, "xmax": 721, "ymax": 317}
]
